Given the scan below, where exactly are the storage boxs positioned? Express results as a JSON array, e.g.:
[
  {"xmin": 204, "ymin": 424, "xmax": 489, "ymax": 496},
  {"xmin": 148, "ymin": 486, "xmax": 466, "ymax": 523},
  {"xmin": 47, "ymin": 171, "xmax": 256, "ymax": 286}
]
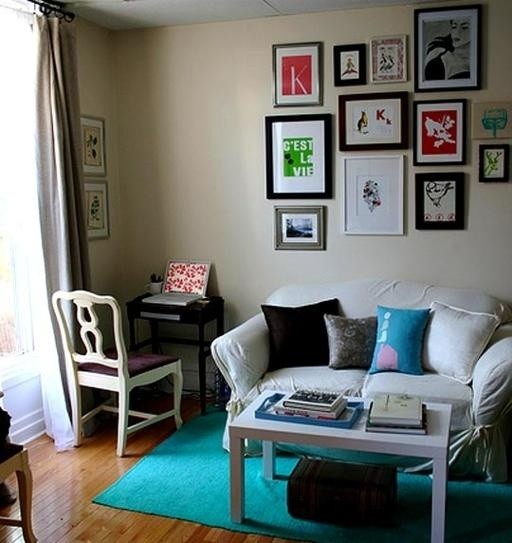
[{"xmin": 286, "ymin": 458, "xmax": 398, "ymax": 527}]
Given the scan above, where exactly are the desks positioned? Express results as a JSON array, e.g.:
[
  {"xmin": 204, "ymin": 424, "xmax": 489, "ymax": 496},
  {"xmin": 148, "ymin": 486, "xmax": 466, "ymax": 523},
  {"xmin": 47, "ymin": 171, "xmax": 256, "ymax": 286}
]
[
  {"xmin": 125, "ymin": 292, "xmax": 224, "ymax": 416},
  {"xmin": 228, "ymin": 389, "xmax": 453, "ymax": 543},
  {"xmin": 0, "ymin": 449, "xmax": 39, "ymax": 543}
]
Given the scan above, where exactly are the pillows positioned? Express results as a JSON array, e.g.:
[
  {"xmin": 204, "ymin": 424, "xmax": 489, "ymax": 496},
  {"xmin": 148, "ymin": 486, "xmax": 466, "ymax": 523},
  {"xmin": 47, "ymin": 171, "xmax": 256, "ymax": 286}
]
[
  {"xmin": 422, "ymin": 300, "xmax": 503, "ymax": 385},
  {"xmin": 261, "ymin": 298, "xmax": 341, "ymax": 370},
  {"xmin": 324, "ymin": 315, "xmax": 377, "ymax": 368},
  {"xmin": 368, "ymin": 305, "xmax": 431, "ymax": 376}
]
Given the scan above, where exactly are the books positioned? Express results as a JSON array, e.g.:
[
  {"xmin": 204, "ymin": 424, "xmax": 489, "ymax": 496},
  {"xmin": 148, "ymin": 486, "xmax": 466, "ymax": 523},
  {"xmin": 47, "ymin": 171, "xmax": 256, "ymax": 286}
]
[{"xmin": 270, "ymin": 386, "xmax": 429, "ymax": 436}]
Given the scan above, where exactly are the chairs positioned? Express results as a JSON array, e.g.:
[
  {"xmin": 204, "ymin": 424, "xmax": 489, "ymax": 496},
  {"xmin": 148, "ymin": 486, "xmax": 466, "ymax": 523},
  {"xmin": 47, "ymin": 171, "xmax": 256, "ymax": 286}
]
[{"xmin": 52, "ymin": 290, "xmax": 184, "ymax": 457}]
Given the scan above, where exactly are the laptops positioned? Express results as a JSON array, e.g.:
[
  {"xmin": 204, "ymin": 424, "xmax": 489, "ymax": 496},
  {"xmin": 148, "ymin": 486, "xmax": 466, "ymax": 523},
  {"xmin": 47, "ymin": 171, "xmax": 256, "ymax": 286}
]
[{"xmin": 142, "ymin": 259, "xmax": 212, "ymax": 307}]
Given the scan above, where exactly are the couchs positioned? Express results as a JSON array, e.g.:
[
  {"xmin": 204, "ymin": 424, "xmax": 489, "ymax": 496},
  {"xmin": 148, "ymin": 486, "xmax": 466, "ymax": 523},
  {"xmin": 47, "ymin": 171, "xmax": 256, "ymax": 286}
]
[{"xmin": 210, "ymin": 281, "xmax": 512, "ymax": 483}]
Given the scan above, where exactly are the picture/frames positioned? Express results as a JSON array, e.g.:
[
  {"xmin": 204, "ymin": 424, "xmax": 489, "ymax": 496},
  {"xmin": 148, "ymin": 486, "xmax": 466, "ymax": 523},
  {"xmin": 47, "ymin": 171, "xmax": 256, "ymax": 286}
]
[
  {"xmin": 79, "ymin": 114, "xmax": 109, "ymax": 238},
  {"xmin": 265, "ymin": 41, "xmax": 333, "ymax": 252}
]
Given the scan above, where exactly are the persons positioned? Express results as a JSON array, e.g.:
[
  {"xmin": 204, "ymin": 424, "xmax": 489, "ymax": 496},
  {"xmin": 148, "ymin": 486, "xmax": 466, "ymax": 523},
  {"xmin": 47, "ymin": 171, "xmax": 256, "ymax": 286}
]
[
  {"xmin": 342, "ymin": 56, "xmax": 359, "ymax": 77},
  {"xmin": 424, "ymin": 15, "xmax": 472, "ymax": 81}
]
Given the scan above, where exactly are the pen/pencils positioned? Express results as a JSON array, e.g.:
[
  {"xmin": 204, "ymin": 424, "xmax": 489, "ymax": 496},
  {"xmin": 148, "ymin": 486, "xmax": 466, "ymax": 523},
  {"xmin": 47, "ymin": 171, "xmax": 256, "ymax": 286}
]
[
  {"xmin": 157, "ymin": 275, "xmax": 161, "ymax": 283},
  {"xmin": 150, "ymin": 273, "xmax": 156, "ymax": 283}
]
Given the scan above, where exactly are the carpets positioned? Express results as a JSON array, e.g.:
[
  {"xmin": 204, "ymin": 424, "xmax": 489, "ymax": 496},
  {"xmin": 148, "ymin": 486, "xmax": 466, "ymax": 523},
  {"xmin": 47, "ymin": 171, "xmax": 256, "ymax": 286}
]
[{"xmin": 92, "ymin": 404, "xmax": 512, "ymax": 543}]
